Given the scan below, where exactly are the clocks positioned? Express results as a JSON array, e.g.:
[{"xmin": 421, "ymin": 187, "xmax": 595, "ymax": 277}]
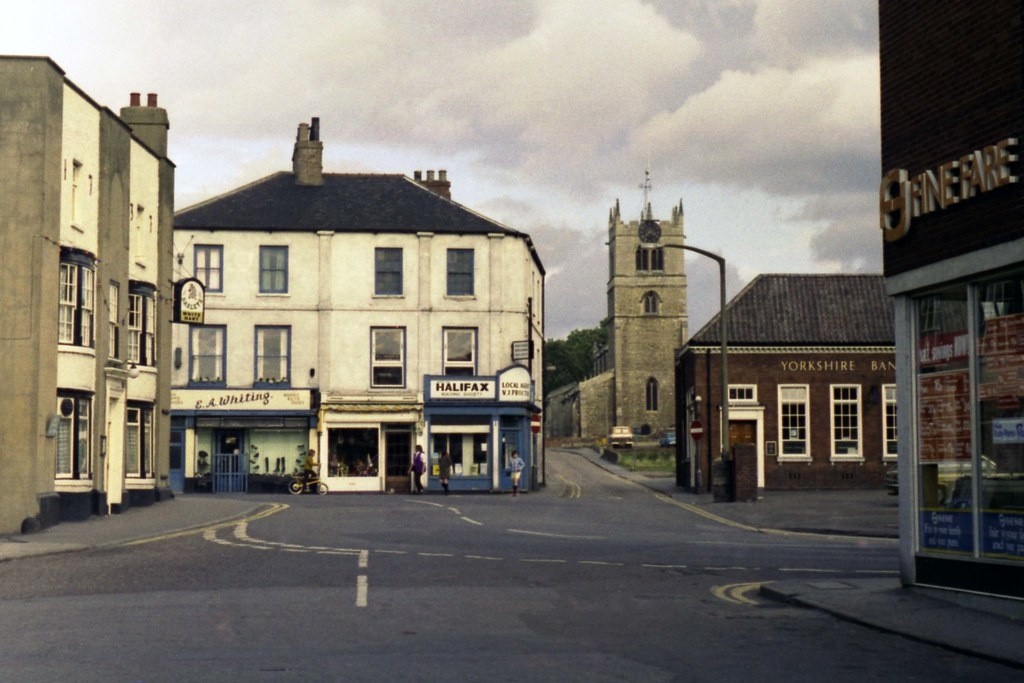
[{"xmin": 638, "ymin": 220, "xmax": 661, "ymax": 243}]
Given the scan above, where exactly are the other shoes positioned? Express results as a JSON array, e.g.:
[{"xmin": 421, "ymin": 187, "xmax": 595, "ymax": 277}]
[{"xmin": 416, "ymin": 488, "xmax": 424, "ymax": 495}]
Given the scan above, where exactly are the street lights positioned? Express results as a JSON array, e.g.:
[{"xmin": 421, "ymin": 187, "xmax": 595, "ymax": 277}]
[{"xmin": 640, "ymin": 240, "xmax": 733, "ymax": 505}]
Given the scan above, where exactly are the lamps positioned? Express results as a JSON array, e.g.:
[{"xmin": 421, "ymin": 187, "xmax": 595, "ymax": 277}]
[{"xmin": 129, "ymin": 363, "xmax": 139, "ymax": 378}]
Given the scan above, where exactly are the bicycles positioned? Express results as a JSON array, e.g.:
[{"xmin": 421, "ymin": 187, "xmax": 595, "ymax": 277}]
[{"xmin": 287, "ymin": 466, "xmax": 329, "ymax": 496}]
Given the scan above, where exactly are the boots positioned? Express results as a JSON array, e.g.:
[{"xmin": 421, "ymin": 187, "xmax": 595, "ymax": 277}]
[
  {"xmin": 513, "ymin": 485, "xmax": 517, "ymax": 496},
  {"xmin": 441, "ymin": 482, "xmax": 449, "ymax": 496}
]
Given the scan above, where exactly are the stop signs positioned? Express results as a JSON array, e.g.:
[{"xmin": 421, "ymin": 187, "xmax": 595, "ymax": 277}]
[
  {"xmin": 529, "ymin": 414, "xmax": 542, "ymax": 434},
  {"xmin": 688, "ymin": 420, "xmax": 704, "ymax": 441}
]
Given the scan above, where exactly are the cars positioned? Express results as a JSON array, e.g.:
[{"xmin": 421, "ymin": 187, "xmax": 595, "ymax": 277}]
[
  {"xmin": 608, "ymin": 427, "xmax": 633, "ymax": 449},
  {"xmin": 660, "ymin": 431, "xmax": 676, "ymax": 449},
  {"xmin": 883, "ymin": 455, "xmax": 999, "ymax": 505}
]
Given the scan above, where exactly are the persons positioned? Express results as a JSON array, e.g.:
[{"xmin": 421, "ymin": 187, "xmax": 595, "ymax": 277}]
[
  {"xmin": 301, "ymin": 450, "xmax": 316, "ymax": 490},
  {"xmin": 508, "ymin": 450, "xmax": 525, "ymax": 497},
  {"xmin": 409, "ymin": 445, "xmax": 427, "ymax": 494},
  {"xmin": 438, "ymin": 445, "xmax": 450, "ymax": 494}
]
